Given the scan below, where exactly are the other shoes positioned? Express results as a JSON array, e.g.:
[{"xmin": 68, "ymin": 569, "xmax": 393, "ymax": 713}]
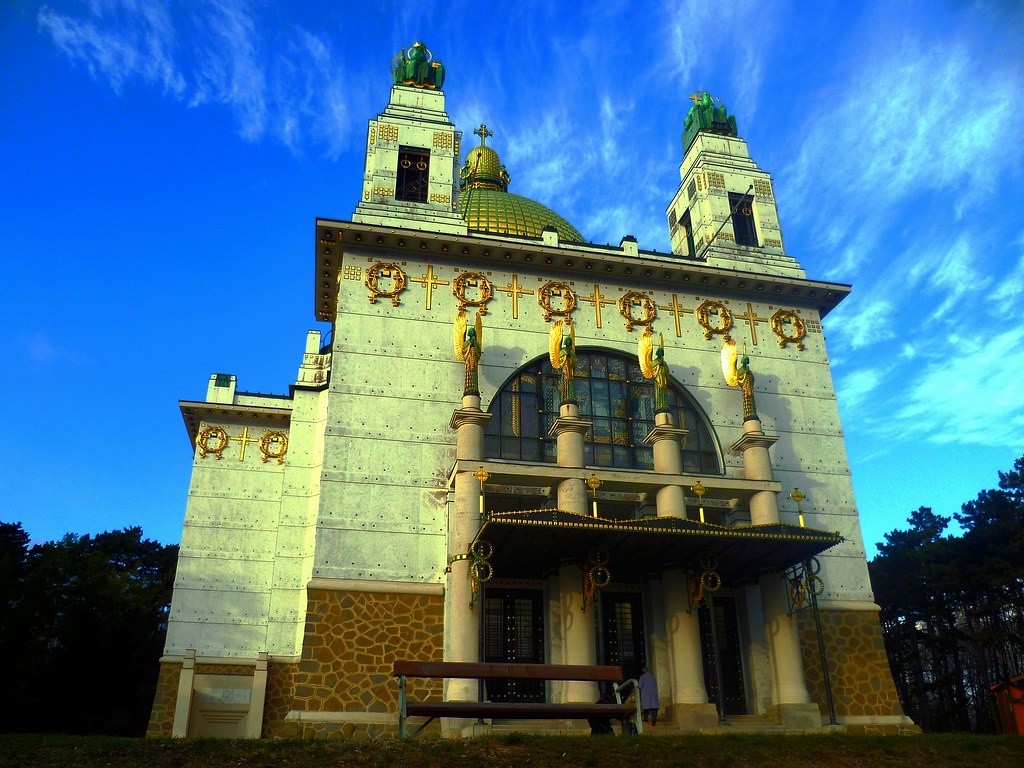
[
  {"xmin": 651, "ymin": 722, "xmax": 655, "ymax": 726},
  {"xmin": 643, "ymin": 718, "xmax": 648, "ymax": 722}
]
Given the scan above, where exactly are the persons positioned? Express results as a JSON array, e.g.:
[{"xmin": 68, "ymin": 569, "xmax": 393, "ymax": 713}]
[
  {"xmin": 736, "ymin": 355, "xmax": 759, "ymax": 419},
  {"xmin": 559, "ymin": 337, "xmax": 578, "ymax": 404},
  {"xmin": 698, "ymin": 92, "xmax": 728, "ymax": 129},
  {"xmin": 403, "ymin": 42, "xmax": 433, "ymax": 84},
  {"xmin": 652, "ymin": 348, "xmax": 669, "ymax": 411},
  {"xmin": 638, "ymin": 667, "xmax": 660, "ymax": 727},
  {"xmin": 462, "ymin": 327, "xmax": 482, "ymax": 395}
]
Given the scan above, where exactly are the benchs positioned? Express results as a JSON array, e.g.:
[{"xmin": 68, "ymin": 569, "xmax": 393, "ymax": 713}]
[{"xmin": 393, "ymin": 659, "xmax": 643, "ymax": 741}]
